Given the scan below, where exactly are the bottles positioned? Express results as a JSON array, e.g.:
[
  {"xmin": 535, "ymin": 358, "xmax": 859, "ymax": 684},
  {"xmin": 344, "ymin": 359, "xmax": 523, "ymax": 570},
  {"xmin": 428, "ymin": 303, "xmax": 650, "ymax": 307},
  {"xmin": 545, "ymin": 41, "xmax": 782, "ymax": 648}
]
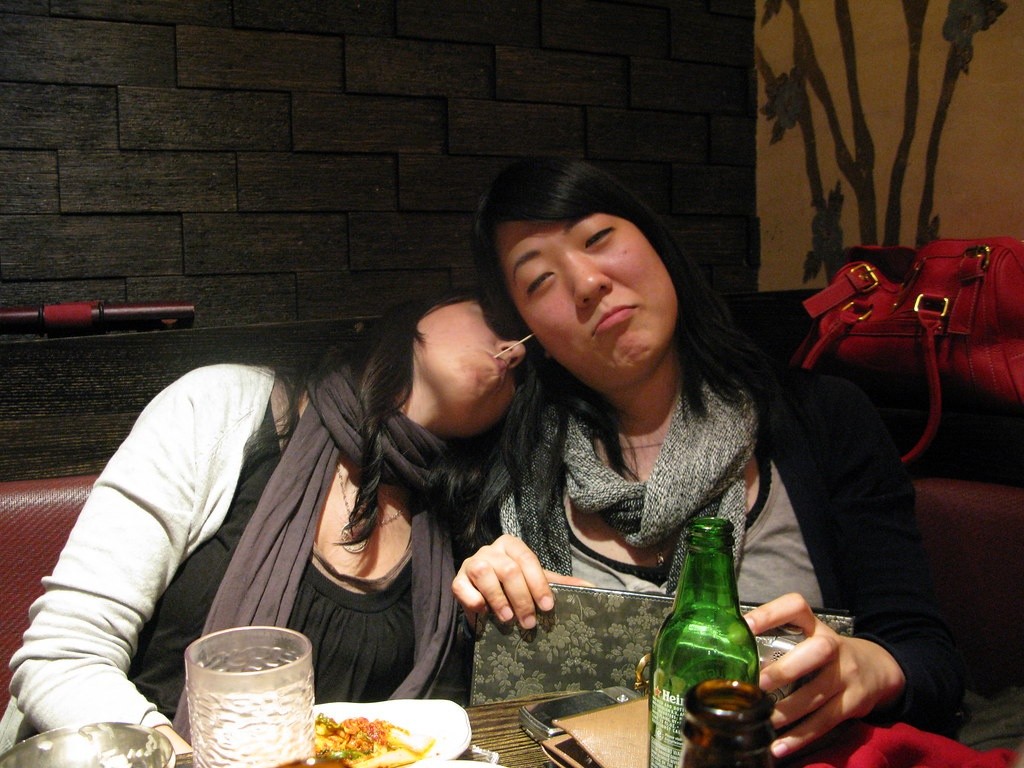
[
  {"xmin": 643, "ymin": 517, "xmax": 762, "ymax": 768},
  {"xmin": 677, "ymin": 677, "xmax": 776, "ymax": 768}
]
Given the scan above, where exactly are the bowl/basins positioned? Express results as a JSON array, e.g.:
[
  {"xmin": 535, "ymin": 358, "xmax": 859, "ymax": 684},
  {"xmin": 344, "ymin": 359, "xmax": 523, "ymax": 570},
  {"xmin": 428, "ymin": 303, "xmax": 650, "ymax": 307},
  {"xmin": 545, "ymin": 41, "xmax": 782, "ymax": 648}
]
[{"xmin": 0, "ymin": 721, "xmax": 178, "ymax": 768}]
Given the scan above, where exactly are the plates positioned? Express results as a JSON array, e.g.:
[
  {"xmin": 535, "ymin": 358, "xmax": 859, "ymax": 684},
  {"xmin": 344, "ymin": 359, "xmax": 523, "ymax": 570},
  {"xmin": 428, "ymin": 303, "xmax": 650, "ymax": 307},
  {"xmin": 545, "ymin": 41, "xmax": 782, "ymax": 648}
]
[
  {"xmin": 311, "ymin": 699, "xmax": 470, "ymax": 768},
  {"xmin": 401, "ymin": 759, "xmax": 511, "ymax": 768}
]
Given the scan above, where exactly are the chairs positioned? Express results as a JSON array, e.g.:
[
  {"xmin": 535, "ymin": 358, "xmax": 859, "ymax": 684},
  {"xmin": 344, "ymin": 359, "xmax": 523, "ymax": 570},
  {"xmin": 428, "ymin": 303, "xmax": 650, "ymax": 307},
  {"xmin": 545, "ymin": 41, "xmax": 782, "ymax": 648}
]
[
  {"xmin": 0, "ymin": 475, "xmax": 98, "ymax": 721},
  {"xmin": 913, "ymin": 478, "xmax": 1024, "ymax": 698}
]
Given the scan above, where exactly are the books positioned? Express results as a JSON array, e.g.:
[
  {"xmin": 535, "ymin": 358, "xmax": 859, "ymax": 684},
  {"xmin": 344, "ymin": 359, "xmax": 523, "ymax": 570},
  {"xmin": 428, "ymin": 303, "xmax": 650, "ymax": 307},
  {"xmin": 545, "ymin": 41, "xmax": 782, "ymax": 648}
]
[{"xmin": 470, "ymin": 582, "xmax": 855, "ymax": 707}]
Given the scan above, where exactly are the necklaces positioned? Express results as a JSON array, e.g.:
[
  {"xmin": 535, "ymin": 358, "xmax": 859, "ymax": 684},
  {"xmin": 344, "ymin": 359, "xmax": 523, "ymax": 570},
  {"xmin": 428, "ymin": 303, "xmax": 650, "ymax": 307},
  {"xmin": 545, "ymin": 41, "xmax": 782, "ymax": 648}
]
[
  {"xmin": 336, "ymin": 461, "xmax": 412, "ymax": 552},
  {"xmin": 655, "ymin": 550, "xmax": 665, "ymax": 565}
]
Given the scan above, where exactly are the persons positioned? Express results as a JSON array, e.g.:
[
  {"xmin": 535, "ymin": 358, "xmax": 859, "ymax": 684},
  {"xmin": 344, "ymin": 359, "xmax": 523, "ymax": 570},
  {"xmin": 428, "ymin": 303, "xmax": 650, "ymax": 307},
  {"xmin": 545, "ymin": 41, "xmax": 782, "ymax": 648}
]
[
  {"xmin": 451, "ymin": 154, "xmax": 969, "ymax": 759},
  {"xmin": 0, "ymin": 288, "xmax": 528, "ymax": 755}
]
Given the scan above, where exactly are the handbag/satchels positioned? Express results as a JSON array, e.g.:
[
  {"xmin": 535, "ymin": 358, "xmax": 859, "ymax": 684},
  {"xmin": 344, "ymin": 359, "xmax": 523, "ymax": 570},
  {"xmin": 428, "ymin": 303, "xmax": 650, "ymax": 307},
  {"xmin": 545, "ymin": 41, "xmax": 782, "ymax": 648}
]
[{"xmin": 790, "ymin": 237, "xmax": 1024, "ymax": 459}]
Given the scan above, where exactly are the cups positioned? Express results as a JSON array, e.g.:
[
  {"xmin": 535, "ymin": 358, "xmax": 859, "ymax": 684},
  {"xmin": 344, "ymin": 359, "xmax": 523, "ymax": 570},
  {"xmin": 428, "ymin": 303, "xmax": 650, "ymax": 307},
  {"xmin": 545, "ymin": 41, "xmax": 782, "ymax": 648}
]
[{"xmin": 185, "ymin": 624, "xmax": 316, "ymax": 768}]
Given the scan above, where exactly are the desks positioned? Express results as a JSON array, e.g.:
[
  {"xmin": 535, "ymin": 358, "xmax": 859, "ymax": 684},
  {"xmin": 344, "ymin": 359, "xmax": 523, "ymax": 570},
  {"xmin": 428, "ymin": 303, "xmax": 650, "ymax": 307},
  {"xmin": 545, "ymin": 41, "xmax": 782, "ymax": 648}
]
[{"xmin": 174, "ymin": 691, "xmax": 582, "ymax": 768}]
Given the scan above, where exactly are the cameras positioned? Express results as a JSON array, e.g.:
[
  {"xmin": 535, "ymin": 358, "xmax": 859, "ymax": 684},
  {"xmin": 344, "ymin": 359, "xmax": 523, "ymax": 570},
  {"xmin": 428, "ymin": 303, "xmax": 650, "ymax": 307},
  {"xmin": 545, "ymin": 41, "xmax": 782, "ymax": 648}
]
[{"xmin": 516, "ymin": 684, "xmax": 641, "ymax": 745}]
